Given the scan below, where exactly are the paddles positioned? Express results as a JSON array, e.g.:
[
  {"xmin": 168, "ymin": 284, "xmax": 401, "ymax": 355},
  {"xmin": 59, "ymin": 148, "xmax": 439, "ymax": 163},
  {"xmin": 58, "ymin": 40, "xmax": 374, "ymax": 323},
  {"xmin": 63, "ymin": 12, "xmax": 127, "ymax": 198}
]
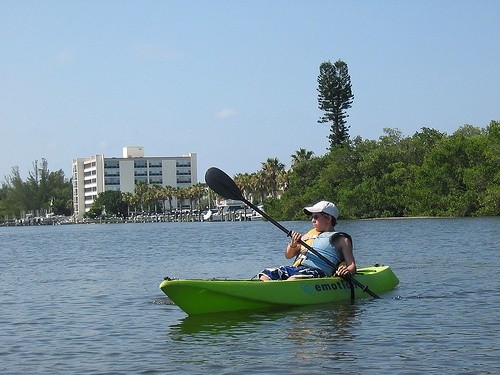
[{"xmin": 205, "ymin": 167, "xmax": 382, "ymax": 298}]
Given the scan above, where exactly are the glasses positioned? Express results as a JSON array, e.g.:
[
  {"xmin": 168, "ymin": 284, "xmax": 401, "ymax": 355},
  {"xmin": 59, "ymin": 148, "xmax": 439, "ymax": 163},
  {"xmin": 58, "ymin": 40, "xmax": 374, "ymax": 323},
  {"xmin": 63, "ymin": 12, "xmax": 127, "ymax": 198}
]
[{"xmin": 309, "ymin": 214, "xmax": 329, "ymax": 219}]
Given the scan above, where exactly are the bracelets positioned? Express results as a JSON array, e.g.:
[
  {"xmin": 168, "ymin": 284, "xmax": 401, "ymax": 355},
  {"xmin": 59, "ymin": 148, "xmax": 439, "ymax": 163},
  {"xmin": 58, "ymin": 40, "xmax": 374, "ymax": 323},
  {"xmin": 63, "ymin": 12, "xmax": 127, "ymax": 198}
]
[{"xmin": 290, "ymin": 244, "xmax": 297, "ymax": 248}]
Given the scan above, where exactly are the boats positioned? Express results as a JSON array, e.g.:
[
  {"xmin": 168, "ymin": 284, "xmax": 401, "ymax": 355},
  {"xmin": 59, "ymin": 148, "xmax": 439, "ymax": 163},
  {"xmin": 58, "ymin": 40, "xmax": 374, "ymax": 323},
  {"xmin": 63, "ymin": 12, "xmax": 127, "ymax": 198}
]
[{"xmin": 159, "ymin": 264, "xmax": 400, "ymax": 317}]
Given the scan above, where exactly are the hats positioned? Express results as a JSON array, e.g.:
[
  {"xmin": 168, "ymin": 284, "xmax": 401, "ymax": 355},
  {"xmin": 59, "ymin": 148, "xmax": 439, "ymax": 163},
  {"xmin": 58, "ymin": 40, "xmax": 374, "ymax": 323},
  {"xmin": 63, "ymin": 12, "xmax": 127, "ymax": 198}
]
[{"xmin": 303, "ymin": 201, "xmax": 338, "ymax": 219}]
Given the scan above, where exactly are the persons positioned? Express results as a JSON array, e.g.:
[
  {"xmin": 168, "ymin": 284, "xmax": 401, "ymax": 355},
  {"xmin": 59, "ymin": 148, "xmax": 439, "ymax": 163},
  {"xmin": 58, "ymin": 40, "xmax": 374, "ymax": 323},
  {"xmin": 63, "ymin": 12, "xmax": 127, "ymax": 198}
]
[{"xmin": 258, "ymin": 201, "xmax": 356, "ymax": 281}]
